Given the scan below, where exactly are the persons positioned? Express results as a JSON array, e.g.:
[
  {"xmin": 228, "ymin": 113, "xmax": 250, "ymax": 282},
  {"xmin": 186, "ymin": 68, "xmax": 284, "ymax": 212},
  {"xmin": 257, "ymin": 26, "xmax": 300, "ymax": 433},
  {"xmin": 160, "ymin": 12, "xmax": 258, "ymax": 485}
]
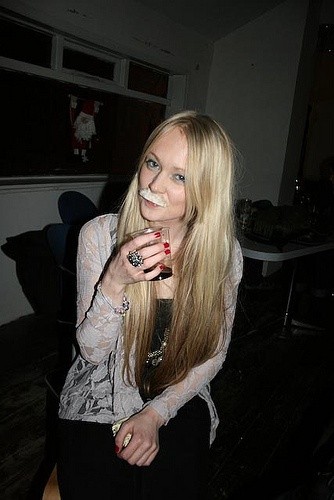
[{"xmin": 54, "ymin": 110, "xmax": 244, "ymax": 500}]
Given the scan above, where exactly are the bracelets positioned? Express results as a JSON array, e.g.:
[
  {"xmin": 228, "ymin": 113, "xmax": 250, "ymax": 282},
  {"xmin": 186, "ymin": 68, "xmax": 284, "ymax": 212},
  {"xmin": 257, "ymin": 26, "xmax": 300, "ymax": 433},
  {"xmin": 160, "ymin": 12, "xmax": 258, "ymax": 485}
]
[{"xmin": 95, "ymin": 280, "xmax": 129, "ymax": 313}]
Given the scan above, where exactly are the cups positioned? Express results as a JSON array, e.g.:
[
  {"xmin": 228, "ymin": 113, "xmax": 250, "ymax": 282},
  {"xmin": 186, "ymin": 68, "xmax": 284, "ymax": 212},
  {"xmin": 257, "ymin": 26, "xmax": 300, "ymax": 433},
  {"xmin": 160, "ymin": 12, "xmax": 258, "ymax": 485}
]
[{"xmin": 128, "ymin": 226, "xmax": 173, "ymax": 282}]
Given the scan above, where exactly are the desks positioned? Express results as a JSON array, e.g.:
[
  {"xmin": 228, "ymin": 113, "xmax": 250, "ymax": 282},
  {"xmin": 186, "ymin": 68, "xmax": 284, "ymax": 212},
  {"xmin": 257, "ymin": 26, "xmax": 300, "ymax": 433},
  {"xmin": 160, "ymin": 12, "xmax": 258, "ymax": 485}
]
[{"xmin": 231, "ymin": 235, "xmax": 334, "ymax": 344}]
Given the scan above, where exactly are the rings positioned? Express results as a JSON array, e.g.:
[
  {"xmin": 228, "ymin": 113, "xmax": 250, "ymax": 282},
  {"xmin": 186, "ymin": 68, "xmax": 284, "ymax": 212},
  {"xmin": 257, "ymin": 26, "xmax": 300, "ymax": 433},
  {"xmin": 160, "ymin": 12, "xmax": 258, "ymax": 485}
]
[{"xmin": 127, "ymin": 250, "xmax": 143, "ymax": 267}]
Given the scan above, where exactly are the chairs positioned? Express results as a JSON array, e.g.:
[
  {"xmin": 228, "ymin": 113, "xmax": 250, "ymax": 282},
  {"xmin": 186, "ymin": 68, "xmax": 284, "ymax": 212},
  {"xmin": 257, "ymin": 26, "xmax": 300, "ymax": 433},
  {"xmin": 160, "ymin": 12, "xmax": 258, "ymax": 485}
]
[{"xmin": 42, "ymin": 223, "xmax": 80, "ymax": 500}]
